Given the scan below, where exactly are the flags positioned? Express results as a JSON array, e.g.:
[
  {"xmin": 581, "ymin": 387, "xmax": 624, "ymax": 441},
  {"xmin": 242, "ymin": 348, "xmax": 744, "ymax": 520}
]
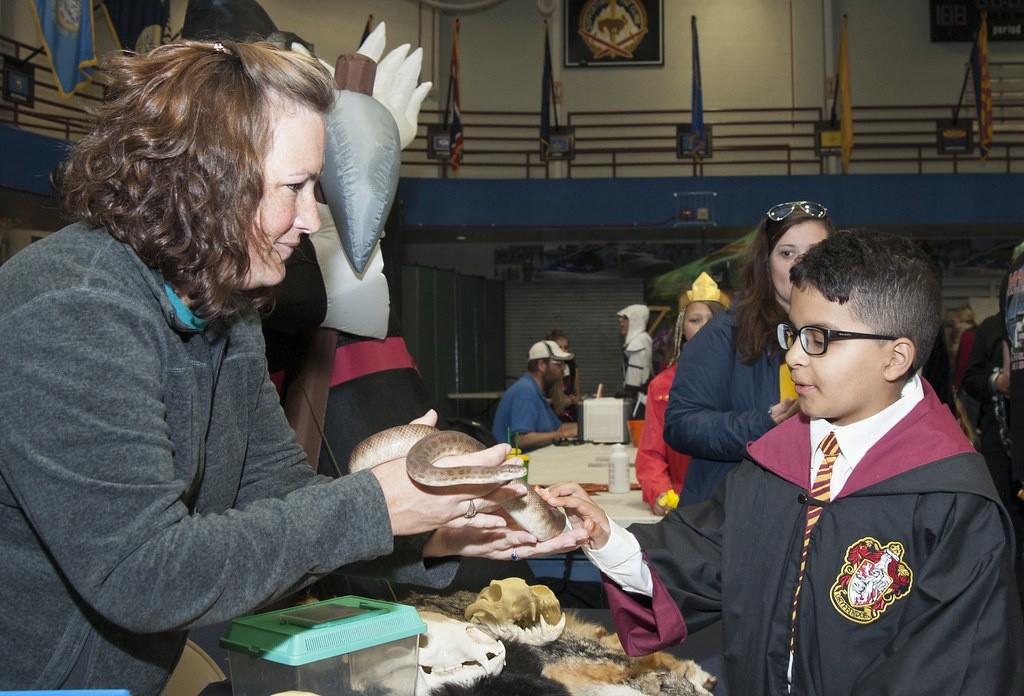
[
  {"xmin": 182, "ymin": 0, "xmax": 278, "ymax": 45},
  {"xmin": 688, "ymin": 18, "xmax": 706, "ymax": 158},
  {"xmin": 100, "ymin": 0, "xmax": 171, "ymax": 58},
  {"xmin": 968, "ymin": 18, "xmax": 991, "ymax": 161},
  {"xmin": 451, "ymin": 28, "xmax": 463, "ymax": 170},
  {"xmin": 29, "ymin": 0, "xmax": 98, "ymax": 101},
  {"xmin": 837, "ymin": 21, "xmax": 852, "ymax": 173},
  {"xmin": 537, "ymin": 30, "xmax": 553, "ymax": 153}
]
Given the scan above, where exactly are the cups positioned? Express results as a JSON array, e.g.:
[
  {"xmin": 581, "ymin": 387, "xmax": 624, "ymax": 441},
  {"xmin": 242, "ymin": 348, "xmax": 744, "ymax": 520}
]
[
  {"xmin": 504, "ymin": 447, "xmax": 529, "ymax": 482},
  {"xmin": 626, "ymin": 420, "xmax": 644, "ymax": 447}
]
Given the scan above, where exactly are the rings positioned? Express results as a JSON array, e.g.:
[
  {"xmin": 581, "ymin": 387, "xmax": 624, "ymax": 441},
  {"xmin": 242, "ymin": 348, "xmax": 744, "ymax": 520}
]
[
  {"xmin": 511, "ymin": 548, "xmax": 518, "ymax": 561},
  {"xmin": 465, "ymin": 500, "xmax": 476, "ymax": 518}
]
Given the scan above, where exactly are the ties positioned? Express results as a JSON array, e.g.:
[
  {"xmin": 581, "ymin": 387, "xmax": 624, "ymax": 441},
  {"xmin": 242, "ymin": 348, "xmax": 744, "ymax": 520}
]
[{"xmin": 790, "ymin": 431, "xmax": 841, "ymax": 651}]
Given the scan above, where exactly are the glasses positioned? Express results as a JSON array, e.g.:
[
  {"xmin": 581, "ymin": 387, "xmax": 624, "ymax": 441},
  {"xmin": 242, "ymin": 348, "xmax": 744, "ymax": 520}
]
[
  {"xmin": 764, "ymin": 202, "xmax": 830, "ymax": 222},
  {"xmin": 777, "ymin": 321, "xmax": 914, "ymax": 375},
  {"xmin": 548, "ymin": 360, "xmax": 563, "ymax": 366}
]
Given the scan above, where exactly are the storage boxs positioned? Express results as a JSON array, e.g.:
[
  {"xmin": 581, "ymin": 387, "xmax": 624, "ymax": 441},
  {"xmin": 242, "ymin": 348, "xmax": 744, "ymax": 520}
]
[
  {"xmin": 215, "ymin": 592, "xmax": 427, "ymax": 696},
  {"xmin": 577, "ymin": 397, "xmax": 628, "ymax": 444}
]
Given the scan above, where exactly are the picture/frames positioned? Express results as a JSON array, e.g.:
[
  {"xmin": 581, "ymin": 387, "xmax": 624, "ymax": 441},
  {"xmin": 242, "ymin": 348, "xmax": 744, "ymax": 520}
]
[{"xmin": 564, "ymin": 0, "xmax": 664, "ymax": 67}]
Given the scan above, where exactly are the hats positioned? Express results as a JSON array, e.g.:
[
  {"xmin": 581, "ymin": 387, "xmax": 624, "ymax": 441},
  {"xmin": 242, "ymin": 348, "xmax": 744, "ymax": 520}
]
[{"xmin": 528, "ymin": 340, "xmax": 574, "ymax": 361}]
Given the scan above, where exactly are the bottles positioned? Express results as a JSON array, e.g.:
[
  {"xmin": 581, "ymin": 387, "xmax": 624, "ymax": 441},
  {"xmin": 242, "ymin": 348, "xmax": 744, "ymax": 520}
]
[{"xmin": 609, "ymin": 444, "xmax": 630, "ymax": 494}]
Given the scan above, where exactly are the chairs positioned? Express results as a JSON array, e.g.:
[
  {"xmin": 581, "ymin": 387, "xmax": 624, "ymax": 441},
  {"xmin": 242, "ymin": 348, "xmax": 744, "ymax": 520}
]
[{"xmin": 450, "ymin": 409, "xmax": 494, "ymax": 446}]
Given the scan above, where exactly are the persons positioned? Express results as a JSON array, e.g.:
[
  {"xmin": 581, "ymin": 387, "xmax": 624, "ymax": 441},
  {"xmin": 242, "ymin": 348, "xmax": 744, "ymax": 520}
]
[
  {"xmin": 492, "ymin": 341, "xmax": 578, "ymax": 452},
  {"xmin": 662, "ymin": 199, "xmax": 837, "ymax": 507},
  {"xmin": 535, "ymin": 227, "xmax": 1018, "ymax": 696},
  {"xmin": 925, "ymin": 233, "xmax": 1024, "ymax": 541},
  {"xmin": 613, "ymin": 304, "xmax": 656, "ymax": 420},
  {"xmin": 635, "ymin": 271, "xmax": 731, "ymax": 515},
  {"xmin": 542, "ymin": 330, "xmax": 576, "ymax": 422},
  {"xmin": 1, "ymin": 41, "xmax": 513, "ymax": 696}
]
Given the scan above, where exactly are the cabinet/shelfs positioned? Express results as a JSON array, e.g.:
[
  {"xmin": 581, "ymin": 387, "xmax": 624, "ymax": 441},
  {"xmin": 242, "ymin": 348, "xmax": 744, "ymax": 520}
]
[{"xmin": 400, "ymin": 262, "xmax": 505, "ymax": 430}]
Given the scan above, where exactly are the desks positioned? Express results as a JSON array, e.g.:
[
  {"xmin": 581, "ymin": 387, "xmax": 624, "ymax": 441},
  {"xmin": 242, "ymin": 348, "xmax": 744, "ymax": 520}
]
[{"xmin": 446, "ymin": 392, "xmax": 504, "ymax": 399}]
